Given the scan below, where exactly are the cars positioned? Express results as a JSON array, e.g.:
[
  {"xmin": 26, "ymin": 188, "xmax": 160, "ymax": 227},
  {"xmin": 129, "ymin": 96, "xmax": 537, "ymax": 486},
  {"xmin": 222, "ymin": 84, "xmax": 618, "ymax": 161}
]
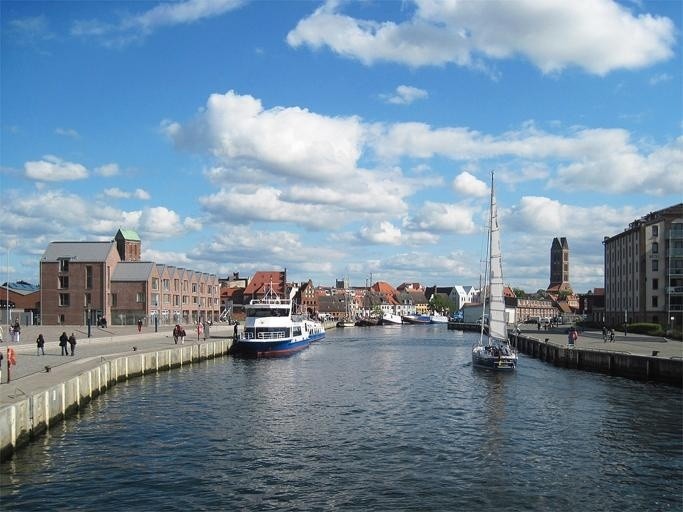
[
  {"xmin": 475, "ymin": 313, "xmax": 487, "ymax": 325},
  {"xmin": 522, "ymin": 316, "xmax": 562, "ymax": 324}
]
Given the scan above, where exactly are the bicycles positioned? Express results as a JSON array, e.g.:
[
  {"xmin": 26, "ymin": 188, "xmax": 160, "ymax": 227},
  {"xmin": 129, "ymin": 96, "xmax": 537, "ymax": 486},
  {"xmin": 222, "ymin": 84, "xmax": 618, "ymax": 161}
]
[
  {"xmin": 607, "ymin": 334, "xmax": 615, "ymax": 344},
  {"xmin": 602, "ymin": 334, "xmax": 606, "ymax": 342}
]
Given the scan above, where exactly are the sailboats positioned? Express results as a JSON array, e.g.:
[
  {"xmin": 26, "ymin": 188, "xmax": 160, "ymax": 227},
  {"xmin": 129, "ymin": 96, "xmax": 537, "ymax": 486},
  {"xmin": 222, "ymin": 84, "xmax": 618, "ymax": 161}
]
[{"xmin": 469, "ymin": 167, "xmax": 518, "ymax": 372}]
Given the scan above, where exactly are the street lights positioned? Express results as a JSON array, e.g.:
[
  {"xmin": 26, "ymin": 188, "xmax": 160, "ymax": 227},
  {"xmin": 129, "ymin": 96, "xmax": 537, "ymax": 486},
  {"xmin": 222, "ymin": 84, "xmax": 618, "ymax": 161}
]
[{"xmin": 669, "ymin": 316, "xmax": 675, "ymax": 337}]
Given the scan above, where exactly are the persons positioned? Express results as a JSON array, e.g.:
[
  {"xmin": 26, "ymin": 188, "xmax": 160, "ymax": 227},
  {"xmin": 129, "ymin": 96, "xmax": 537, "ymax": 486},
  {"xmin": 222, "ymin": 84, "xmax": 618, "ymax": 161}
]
[
  {"xmin": 137, "ymin": 319, "xmax": 142, "ymax": 333},
  {"xmin": 35, "ymin": 333, "xmax": 44, "ymax": 356},
  {"xmin": 196, "ymin": 321, "xmax": 203, "ymax": 337},
  {"xmin": 567, "ymin": 326, "xmax": 579, "ymax": 344},
  {"xmin": 100, "ymin": 315, "xmax": 106, "ymax": 328},
  {"xmin": 487, "ymin": 344, "xmax": 508, "ymax": 356},
  {"xmin": 202, "ymin": 321, "xmax": 209, "ymax": 338},
  {"xmin": 232, "ymin": 321, "xmax": 237, "ymax": 338},
  {"xmin": 8, "ymin": 316, "xmax": 21, "ymax": 342},
  {"xmin": 67, "ymin": 332, "xmax": 76, "ymax": 356},
  {"xmin": 172, "ymin": 324, "xmax": 185, "ymax": 344},
  {"xmin": 59, "ymin": 331, "xmax": 69, "ymax": 356},
  {"xmin": 0, "ymin": 351, "xmax": 3, "ymax": 370},
  {"xmin": 602, "ymin": 325, "xmax": 616, "ymax": 344}
]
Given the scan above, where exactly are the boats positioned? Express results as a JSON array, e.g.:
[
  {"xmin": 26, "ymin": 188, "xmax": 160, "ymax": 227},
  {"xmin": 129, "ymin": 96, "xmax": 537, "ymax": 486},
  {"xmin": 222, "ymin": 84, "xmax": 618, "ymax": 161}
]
[
  {"xmin": 228, "ymin": 276, "xmax": 447, "ymax": 359},
  {"xmin": 449, "ymin": 310, "xmax": 463, "ymax": 323}
]
